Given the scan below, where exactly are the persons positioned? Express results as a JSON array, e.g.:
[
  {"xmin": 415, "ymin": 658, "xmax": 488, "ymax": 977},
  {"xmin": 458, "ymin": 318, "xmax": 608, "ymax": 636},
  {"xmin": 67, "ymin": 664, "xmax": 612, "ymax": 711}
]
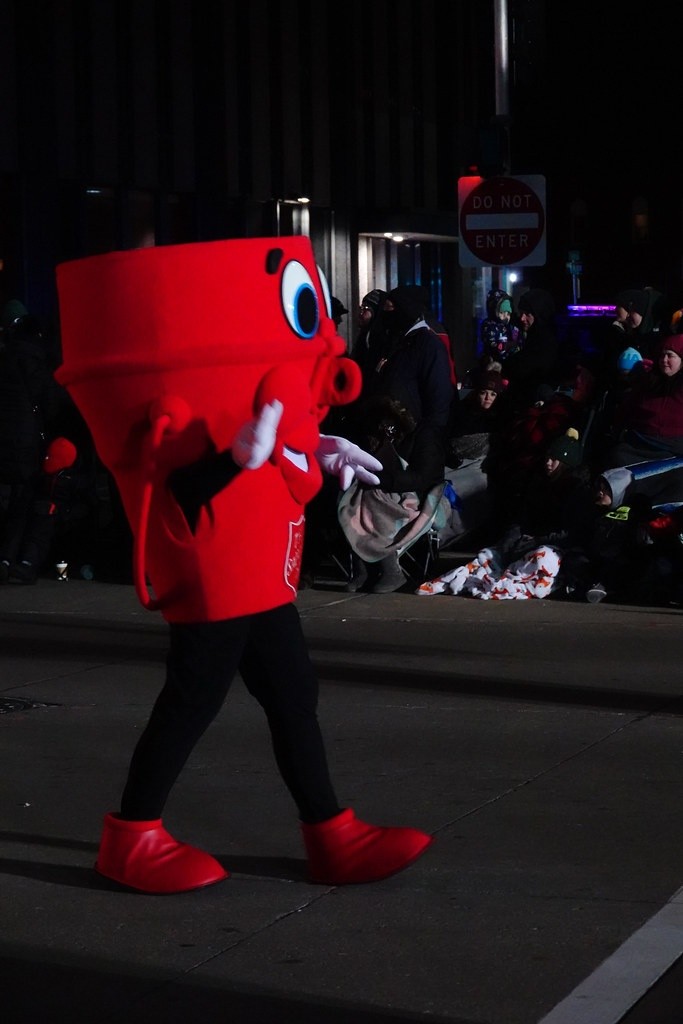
[
  {"xmin": 338, "ymin": 286, "xmax": 682, "ymax": 607},
  {"xmin": 0, "ymin": 298, "xmax": 79, "ymax": 584}
]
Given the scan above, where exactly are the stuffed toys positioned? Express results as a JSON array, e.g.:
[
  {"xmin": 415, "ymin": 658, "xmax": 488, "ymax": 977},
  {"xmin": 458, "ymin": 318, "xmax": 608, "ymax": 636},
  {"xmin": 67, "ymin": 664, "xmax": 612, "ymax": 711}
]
[{"xmin": 53, "ymin": 236, "xmax": 434, "ymax": 890}]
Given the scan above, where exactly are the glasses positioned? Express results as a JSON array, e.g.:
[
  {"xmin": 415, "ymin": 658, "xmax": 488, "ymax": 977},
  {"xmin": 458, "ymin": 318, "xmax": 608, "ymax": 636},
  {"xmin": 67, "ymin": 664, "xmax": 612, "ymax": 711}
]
[{"xmin": 359, "ymin": 305, "xmax": 371, "ymax": 312}]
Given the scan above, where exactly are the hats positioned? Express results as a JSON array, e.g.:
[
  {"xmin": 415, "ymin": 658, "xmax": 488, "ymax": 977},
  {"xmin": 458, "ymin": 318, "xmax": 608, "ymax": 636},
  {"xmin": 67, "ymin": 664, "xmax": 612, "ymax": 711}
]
[
  {"xmin": 662, "ymin": 335, "xmax": 683, "ymax": 359},
  {"xmin": 628, "ymin": 291, "xmax": 649, "ymax": 317},
  {"xmin": 500, "ymin": 299, "xmax": 513, "ymax": 313},
  {"xmin": 617, "ymin": 290, "xmax": 636, "ymax": 312},
  {"xmin": 549, "ymin": 428, "xmax": 579, "ymax": 466},
  {"xmin": 617, "ymin": 346, "xmax": 643, "ymax": 370},
  {"xmin": 475, "ymin": 374, "xmax": 500, "ymax": 395},
  {"xmin": 358, "ymin": 289, "xmax": 388, "ymax": 329},
  {"xmin": 381, "ymin": 284, "xmax": 427, "ymax": 330},
  {"xmin": 42, "ymin": 437, "xmax": 78, "ymax": 475},
  {"xmin": 517, "ymin": 294, "xmax": 533, "ymax": 314}
]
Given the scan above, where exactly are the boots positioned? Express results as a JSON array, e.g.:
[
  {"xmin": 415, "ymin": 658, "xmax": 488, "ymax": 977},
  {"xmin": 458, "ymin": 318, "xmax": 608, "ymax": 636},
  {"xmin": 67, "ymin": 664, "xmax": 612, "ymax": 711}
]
[
  {"xmin": 374, "ymin": 551, "xmax": 407, "ymax": 593},
  {"xmin": 344, "ymin": 553, "xmax": 369, "ymax": 591}
]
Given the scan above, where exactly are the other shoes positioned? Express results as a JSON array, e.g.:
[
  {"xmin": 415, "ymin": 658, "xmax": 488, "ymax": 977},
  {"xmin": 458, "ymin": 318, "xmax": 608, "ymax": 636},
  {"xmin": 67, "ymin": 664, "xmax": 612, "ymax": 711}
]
[
  {"xmin": 586, "ymin": 583, "xmax": 608, "ymax": 603},
  {"xmin": 298, "ymin": 574, "xmax": 314, "ymax": 589},
  {"xmin": 0, "ymin": 562, "xmax": 10, "ymax": 584},
  {"xmin": 9, "ymin": 562, "xmax": 38, "ymax": 584}
]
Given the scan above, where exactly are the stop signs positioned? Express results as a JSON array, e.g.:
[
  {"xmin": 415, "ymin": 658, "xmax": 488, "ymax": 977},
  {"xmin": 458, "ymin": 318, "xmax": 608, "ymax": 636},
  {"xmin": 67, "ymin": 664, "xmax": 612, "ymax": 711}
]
[{"xmin": 454, "ymin": 174, "xmax": 548, "ymax": 270}]
